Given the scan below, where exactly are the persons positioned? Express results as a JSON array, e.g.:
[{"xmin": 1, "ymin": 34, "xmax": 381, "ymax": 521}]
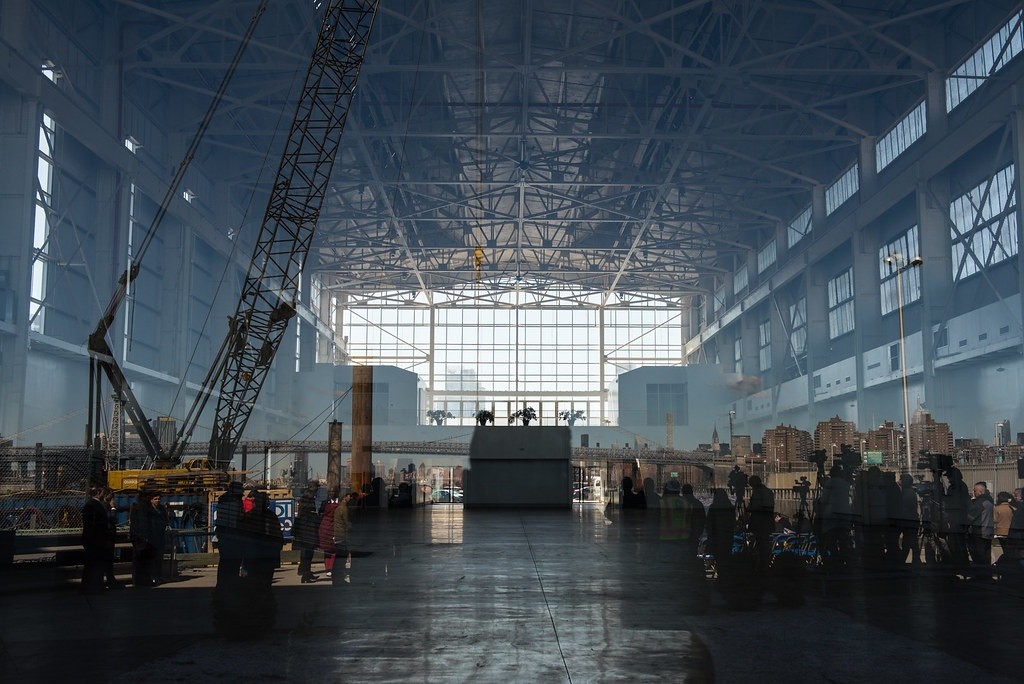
[
  {"xmin": 607, "ymin": 445, "xmax": 1024, "ymax": 595},
  {"xmin": 0, "ymin": 456, "xmax": 442, "ymax": 630}
]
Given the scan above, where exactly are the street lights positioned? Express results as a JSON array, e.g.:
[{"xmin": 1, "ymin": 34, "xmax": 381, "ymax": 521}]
[{"xmin": 882, "ymin": 252, "xmax": 924, "ymax": 479}]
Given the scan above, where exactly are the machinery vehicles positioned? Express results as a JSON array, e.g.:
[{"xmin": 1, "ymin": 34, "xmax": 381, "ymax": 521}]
[{"xmin": 82, "ymin": 0, "xmax": 383, "ymax": 497}]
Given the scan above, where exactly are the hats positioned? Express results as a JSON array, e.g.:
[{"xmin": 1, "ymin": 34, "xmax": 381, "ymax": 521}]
[
  {"xmin": 665, "ymin": 480, "xmax": 680, "ymax": 491},
  {"xmin": 682, "ymin": 484, "xmax": 693, "ymax": 494},
  {"xmin": 308, "ymin": 480, "xmax": 320, "ymax": 487}
]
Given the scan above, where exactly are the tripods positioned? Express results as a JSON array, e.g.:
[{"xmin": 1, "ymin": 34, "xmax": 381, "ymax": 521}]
[
  {"xmin": 734, "ymin": 496, "xmax": 752, "ymax": 553},
  {"xmin": 792, "ymin": 501, "xmax": 812, "ymax": 561}
]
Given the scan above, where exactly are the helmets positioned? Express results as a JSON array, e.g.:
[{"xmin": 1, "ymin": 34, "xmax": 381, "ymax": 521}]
[{"xmin": 339, "ymin": 488, "xmax": 353, "ymax": 500}]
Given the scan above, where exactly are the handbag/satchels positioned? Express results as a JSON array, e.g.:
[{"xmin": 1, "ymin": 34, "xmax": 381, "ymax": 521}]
[
  {"xmin": 696, "ymin": 526, "xmax": 714, "ymax": 558},
  {"xmin": 290, "ymin": 517, "xmax": 302, "ymax": 536}
]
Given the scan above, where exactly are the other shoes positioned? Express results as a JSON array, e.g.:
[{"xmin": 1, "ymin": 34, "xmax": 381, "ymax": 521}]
[
  {"xmin": 309, "ymin": 573, "xmax": 320, "ymax": 579},
  {"xmin": 332, "ymin": 580, "xmax": 350, "ymax": 586},
  {"xmin": 298, "ymin": 569, "xmax": 317, "ymax": 575},
  {"xmin": 301, "ymin": 577, "xmax": 316, "ymax": 583}
]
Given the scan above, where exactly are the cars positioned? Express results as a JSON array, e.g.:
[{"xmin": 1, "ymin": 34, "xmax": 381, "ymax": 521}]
[
  {"xmin": 574, "ymin": 486, "xmax": 590, "ymax": 499},
  {"xmin": 431, "ymin": 489, "xmax": 463, "ymax": 503}
]
